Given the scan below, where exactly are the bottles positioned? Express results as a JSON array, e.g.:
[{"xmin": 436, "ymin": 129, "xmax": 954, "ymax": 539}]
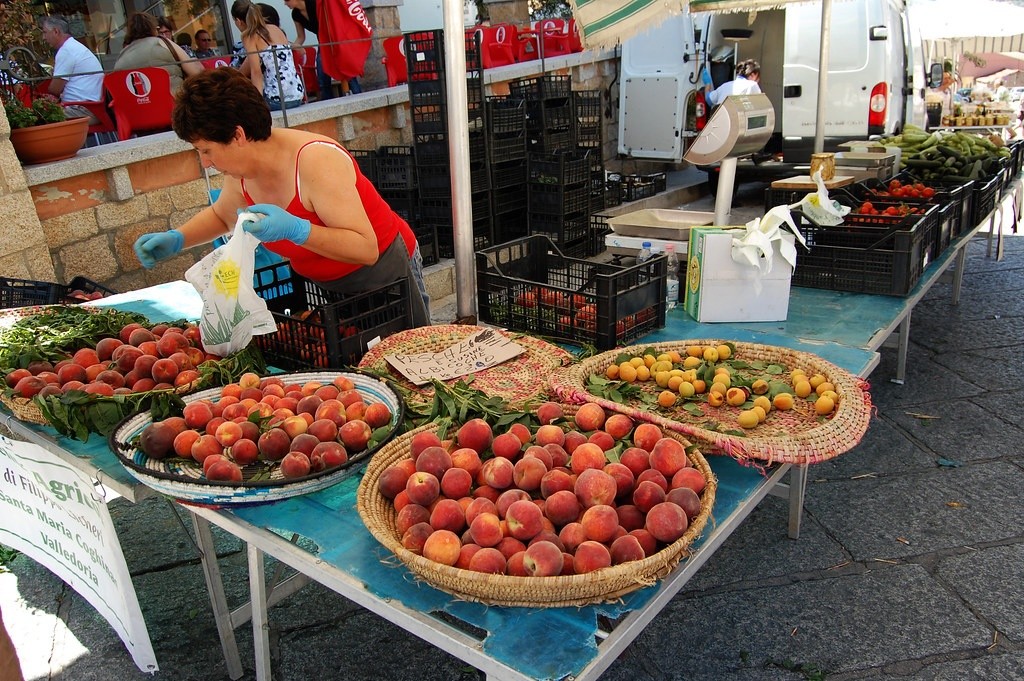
[
  {"xmin": 659, "ymin": 245, "xmax": 678, "ymax": 313},
  {"xmin": 943, "ymin": 115, "xmax": 955, "ymax": 126},
  {"xmin": 810, "ymin": 153, "xmax": 835, "ymax": 181},
  {"xmin": 636, "ymin": 242, "xmax": 654, "ymax": 285}
]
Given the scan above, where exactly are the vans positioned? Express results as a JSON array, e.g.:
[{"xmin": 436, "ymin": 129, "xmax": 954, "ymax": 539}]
[{"xmin": 613, "ymin": 0, "xmax": 944, "ymax": 195}]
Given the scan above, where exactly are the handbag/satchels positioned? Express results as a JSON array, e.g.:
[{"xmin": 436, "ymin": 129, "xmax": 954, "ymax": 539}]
[{"xmin": 185, "ymin": 212, "xmax": 277, "ymax": 356}]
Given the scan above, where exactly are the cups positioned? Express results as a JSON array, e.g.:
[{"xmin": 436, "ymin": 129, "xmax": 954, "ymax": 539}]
[{"xmin": 957, "ymin": 115, "xmax": 1009, "ymax": 126}]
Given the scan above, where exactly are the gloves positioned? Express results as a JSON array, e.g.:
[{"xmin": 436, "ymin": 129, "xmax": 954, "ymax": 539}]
[
  {"xmin": 237, "ymin": 203, "xmax": 310, "ymax": 246},
  {"xmin": 133, "ymin": 230, "xmax": 183, "ymax": 269}
]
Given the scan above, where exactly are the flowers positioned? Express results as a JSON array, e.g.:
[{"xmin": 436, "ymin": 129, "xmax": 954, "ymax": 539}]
[{"xmin": 5, "ymin": 94, "xmax": 66, "ymax": 130}]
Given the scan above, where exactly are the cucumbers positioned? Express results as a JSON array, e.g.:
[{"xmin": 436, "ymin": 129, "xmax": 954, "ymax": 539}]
[{"xmin": 902, "ymin": 146, "xmax": 993, "ymax": 183}]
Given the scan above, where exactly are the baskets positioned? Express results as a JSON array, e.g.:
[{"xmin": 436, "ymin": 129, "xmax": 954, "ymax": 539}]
[
  {"xmin": 780, "ymin": 140, "xmax": 1024, "ymax": 295},
  {"xmin": 0, "ymin": 277, "xmax": 65, "ymax": 309},
  {"xmin": 346, "ymin": 30, "xmax": 668, "ymax": 270},
  {"xmin": 245, "ymin": 261, "xmax": 413, "ymax": 373},
  {"xmin": 475, "ymin": 235, "xmax": 669, "ymax": 355}
]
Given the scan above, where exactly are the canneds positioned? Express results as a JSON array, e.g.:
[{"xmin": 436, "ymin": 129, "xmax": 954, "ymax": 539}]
[
  {"xmin": 942, "ymin": 115, "xmax": 1010, "ymax": 126},
  {"xmin": 810, "ymin": 152, "xmax": 835, "ymax": 181}
]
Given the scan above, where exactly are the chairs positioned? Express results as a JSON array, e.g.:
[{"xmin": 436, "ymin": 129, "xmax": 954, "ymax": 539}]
[{"xmin": 6, "ymin": 19, "xmax": 587, "ymax": 141}]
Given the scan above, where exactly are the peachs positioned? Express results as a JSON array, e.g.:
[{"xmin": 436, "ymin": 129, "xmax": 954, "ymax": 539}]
[
  {"xmin": 379, "ymin": 402, "xmax": 706, "ymax": 577},
  {"xmin": 257, "ymin": 311, "xmax": 361, "ymax": 366},
  {"xmin": 518, "ymin": 288, "xmax": 634, "ymax": 336},
  {"xmin": 65, "ymin": 290, "xmax": 102, "ymax": 304},
  {"xmin": 5, "ymin": 323, "xmax": 222, "ymax": 396},
  {"xmin": 140, "ymin": 373, "xmax": 389, "ymax": 483}
]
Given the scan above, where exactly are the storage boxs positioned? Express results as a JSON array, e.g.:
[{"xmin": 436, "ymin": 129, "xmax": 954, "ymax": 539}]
[
  {"xmin": 0, "ymin": 276, "xmax": 117, "ymax": 310},
  {"xmin": 346, "ymin": 30, "xmax": 666, "ymax": 270},
  {"xmin": 475, "ymin": 234, "xmax": 669, "ymax": 351},
  {"xmin": 683, "ymin": 224, "xmax": 796, "ymax": 322},
  {"xmin": 254, "ymin": 261, "xmax": 414, "ymax": 372},
  {"xmin": 676, "ymin": 140, "xmax": 1024, "ymax": 324}
]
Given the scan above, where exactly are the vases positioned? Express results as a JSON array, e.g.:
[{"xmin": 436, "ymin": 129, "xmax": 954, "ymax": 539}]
[{"xmin": 10, "ymin": 116, "xmax": 91, "ymax": 164}]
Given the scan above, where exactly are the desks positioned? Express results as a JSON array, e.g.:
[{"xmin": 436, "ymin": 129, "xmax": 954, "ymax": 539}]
[{"xmin": 0, "ymin": 184, "xmax": 1018, "ymax": 681}]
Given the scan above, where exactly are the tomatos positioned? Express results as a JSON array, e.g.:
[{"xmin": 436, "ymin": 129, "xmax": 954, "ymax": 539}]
[{"xmin": 847, "ymin": 180, "xmax": 934, "ymax": 224}]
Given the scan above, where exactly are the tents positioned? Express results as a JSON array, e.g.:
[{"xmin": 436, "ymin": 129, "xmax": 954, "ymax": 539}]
[{"xmin": 909, "ymin": 0, "xmax": 1024, "ymax": 119}]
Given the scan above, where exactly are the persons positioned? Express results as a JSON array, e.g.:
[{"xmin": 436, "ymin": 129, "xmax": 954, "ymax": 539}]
[
  {"xmin": 41, "ymin": 16, "xmax": 106, "ymax": 126},
  {"xmin": 994, "ymin": 80, "xmax": 1017, "ymax": 139},
  {"xmin": 155, "ymin": 16, "xmax": 172, "ymax": 41},
  {"xmin": 193, "ymin": 30, "xmax": 223, "ymax": 59},
  {"xmin": 176, "ymin": 32, "xmax": 195, "ymax": 58},
  {"xmin": 702, "ymin": 58, "xmax": 771, "ymax": 209},
  {"xmin": 284, "ymin": 0, "xmax": 362, "ymax": 100},
  {"xmin": 134, "ymin": 66, "xmax": 432, "ymax": 319},
  {"xmin": 113, "ymin": 13, "xmax": 204, "ymax": 98},
  {"xmin": 226, "ymin": 0, "xmax": 304, "ymax": 111}
]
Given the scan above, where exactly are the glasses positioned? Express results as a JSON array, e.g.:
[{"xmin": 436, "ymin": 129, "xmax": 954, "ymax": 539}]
[
  {"xmin": 156, "ymin": 25, "xmax": 161, "ymax": 31},
  {"xmin": 158, "ymin": 30, "xmax": 170, "ymax": 35},
  {"xmin": 198, "ymin": 39, "xmax": 212, "ymax": 42}
]
